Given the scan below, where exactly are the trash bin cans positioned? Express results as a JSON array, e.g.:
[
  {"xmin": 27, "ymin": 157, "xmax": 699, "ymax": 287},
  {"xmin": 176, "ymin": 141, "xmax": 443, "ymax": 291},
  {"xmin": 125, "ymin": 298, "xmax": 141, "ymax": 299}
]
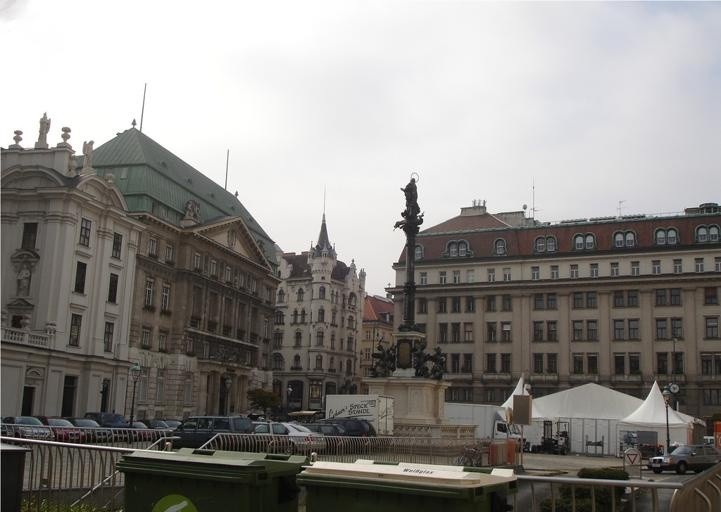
[{"xmin": 537, "ymin": 445, "xmax": 542, "ymax": 452}]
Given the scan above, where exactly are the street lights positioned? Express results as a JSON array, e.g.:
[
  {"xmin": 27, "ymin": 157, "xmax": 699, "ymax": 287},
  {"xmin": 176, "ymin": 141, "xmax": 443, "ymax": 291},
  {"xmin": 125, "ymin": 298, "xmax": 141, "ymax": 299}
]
[
  {"xmin": 286, "ymin": 384, "xmax": 293, "ymax": 423},
  {"xmin": 661, "ymin": 384, "xmax": 673, "ymax": 455},
  {"xmin": 225, "ymin": 375, "xmax": 233, "ymax": 417},
  {"xmin": 129, "ymin": 364, "xmax": 144, "ymax": 427}
]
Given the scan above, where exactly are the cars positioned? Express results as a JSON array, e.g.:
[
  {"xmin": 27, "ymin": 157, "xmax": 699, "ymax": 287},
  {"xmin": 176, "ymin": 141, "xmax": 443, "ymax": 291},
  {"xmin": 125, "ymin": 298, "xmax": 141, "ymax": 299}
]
[{"xmin": 646, "ymin": 444, "xmax": 720, "ymax": 474}]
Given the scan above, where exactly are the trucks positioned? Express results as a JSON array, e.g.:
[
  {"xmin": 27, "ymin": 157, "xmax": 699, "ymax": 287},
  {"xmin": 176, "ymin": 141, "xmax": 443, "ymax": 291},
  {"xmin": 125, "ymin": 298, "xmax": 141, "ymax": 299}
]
[{"xmin": 443, "ymin": 402, "xmax": 526, "ymax": 450}]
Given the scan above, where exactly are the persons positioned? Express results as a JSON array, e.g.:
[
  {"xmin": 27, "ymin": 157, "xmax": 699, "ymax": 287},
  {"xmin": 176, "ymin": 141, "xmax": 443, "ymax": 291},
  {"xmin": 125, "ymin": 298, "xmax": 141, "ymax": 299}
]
[
  {"xmin": 16, "ymin": 264, "xmax": 30, "ymax": 297},
  {"xmin": 540, "ymin": 432, "xmax": 556, "ymax": 454}
]
